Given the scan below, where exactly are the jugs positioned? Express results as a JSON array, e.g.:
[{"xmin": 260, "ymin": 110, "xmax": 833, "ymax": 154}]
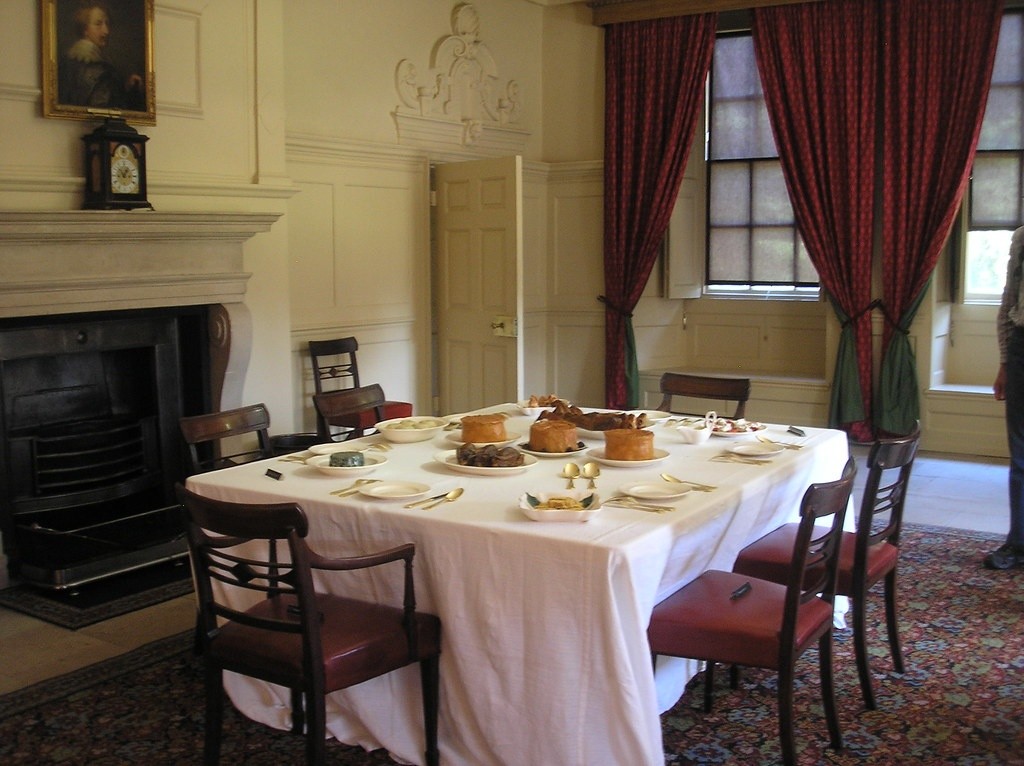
[{"xmin": 676, "ymin": 420, "xmax": 713, "ymax": 445}]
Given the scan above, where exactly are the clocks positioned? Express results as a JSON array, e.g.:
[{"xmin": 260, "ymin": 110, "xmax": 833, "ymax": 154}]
[{"xmin": 80, "ymin": 118, "xmax": 156, "ymax": 212}]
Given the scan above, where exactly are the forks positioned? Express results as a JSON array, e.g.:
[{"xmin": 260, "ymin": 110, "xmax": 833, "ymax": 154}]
[
  {"xmin": 664, "ymin": 418, "xmax": 702, "ymax": 426},
  {"xmin": 328, "ymin": 478, "xmax": 367, "ymax": 495},
  {"xmin": 708, "ymin": 454, "xmax": 768, "ymax": 467},
  {"xmin": 603, "ymin": 495, "xmax": 675, "ymax": 512}
]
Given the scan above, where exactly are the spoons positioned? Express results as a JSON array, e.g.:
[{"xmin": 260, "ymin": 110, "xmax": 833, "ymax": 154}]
[
  {"xmin": 755, "ymin": 435, "xmax": 804, "ymax": 448},
  {"xmin": 421, "ymin": 487, "xmax": 463, "ymax": 511},
  {"xmin": 560, "ymin": 462, "xmax": 581, "ymax": 491},
  {"xmin": 659, "ymin": 472, "xmax": 717, "ymax": 493},
  {"xmin": 581, "ymin": 463, "xmax": 601, "ymax": 490}
]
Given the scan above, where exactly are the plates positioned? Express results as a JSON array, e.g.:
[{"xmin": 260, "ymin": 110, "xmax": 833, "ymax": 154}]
[
  {"xmin": 724, "ymin": 441, "xmax": 786, "ymax": 455},
  {"xmin": 305, "ymin": 453, "xmax": 388, "ymax": 475},
  {"xmin": 433, "ymin": 449, "xmax": 538, "ymax": 475},
  {"xmin": 357, "ymin": 480, "xmax": 432, "ymax": 500},
  {"xmin": 444, "ymin": 432, "xmax": 522, "ymax": 449},
  {"xmin": 309, "ymin": 443, "xmax": 371, "ymax": 454},
  {"xmin": 622, "ymin": 410, "xmax": 672, "ymax": 424},
  {"xmin": 574, "ymin": 421, "xmax": 658, "ymax": 440},
  {"xmin": 520, "ymin": 407, "xmax": 556, "ymax": 416},
  {"xmin": 585, "ymin": 447, "xmax": 671, "ymax": 468},
  {"xmin": 712, "ymin": 424, "xmax": 768, "ymax": 437},
  {"xmin": 515, "ymin": 441, "xmax": 589, "ymax": 457},
  {"xmin": 620, "ymin": 480, "xmax": 692, "ymax": 500},
  {"xmin": 518, "ymin": 489, "xmax": 603, "ymax": 522}
]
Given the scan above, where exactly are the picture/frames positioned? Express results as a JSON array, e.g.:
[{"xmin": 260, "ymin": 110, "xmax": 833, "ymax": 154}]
[{"xmin": 41, "ymin": 0, "xmax": 158, "ymax": 128}]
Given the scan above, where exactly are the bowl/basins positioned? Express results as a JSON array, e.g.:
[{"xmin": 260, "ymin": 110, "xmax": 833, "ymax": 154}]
[{"xmin": 374, "ymin": 415, "xmax": 451, "ymax": 444}]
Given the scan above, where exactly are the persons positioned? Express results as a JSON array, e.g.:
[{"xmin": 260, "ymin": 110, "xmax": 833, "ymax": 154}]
[{"xmin": 984, "ymin": 226, "xmax": 1024, "ymax": 569}]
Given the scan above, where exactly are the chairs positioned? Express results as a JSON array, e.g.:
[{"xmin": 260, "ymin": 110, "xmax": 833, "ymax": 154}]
[
  {"xmin": 730, "ymin": 420, "xmax": 919, "ymax": 711},
  {"xmin": 309, "ymin": 336, "xmax": 412, "ymax": 439},
  {"xmin": 654, "ymin": 372, "xmax": 751, "ymax": 420},
  {"xmin": 179, "ymin": 403, "xmax": 274, "ymax": 474},
  {"xmin": 173, "ymin": 481, "xmax": 443, "ymax": 766},
  {"xmin": 312, "ymin": 384, "xmax": 386, "ymax": 443},
  {"xmin": 646, "ymin": 456, "xmax": 858, "ymax": 766}
]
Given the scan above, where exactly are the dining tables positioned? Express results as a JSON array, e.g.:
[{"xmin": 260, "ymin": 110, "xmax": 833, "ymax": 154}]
[{"xmin": 183, "ymin": 395, "xmax": 852, "ymax": 766}]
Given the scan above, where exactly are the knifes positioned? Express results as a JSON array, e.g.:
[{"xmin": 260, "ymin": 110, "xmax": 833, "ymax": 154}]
[{"xmin": 405, "ymin": 493, "xmax": 448, "ymax": 509}]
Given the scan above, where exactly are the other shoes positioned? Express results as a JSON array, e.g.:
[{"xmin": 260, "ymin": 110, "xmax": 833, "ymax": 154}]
[{"xmin": 983, "ymin": 544, "xmax": 1023, "ymax": 569}]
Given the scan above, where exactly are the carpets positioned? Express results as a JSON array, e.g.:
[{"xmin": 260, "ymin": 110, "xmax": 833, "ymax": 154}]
[{"xmin": 1, "ymin": 515, "xmax": 1023, "ymax": 766}]
